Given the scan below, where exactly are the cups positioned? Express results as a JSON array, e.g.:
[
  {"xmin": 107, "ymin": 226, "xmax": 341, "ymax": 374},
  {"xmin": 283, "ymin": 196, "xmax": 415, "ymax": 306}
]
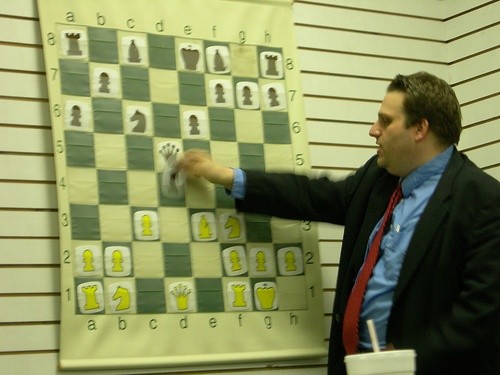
[{"xmin": 343, "ymin": 349, "xmax": 418, "ymax": 375}]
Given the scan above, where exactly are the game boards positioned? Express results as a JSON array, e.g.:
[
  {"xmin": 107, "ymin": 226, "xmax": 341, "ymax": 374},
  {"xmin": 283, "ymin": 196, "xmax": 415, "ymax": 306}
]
[{"xmin": 53, "ymin": 21, "xmax": 308, "ymax": 313}]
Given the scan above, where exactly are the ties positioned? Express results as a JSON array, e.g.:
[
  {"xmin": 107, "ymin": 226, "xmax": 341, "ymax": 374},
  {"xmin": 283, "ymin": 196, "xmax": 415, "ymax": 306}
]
[{"xmin": 341, "ymin": 182, "xmax": 405, "ymax": 356}]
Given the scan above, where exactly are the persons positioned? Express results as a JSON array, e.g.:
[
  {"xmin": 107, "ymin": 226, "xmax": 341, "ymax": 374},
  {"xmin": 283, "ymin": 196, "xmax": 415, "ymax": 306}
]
[{"xmin": 178, "ymin": 70, "xmax": 500, "ymax": 375}]
